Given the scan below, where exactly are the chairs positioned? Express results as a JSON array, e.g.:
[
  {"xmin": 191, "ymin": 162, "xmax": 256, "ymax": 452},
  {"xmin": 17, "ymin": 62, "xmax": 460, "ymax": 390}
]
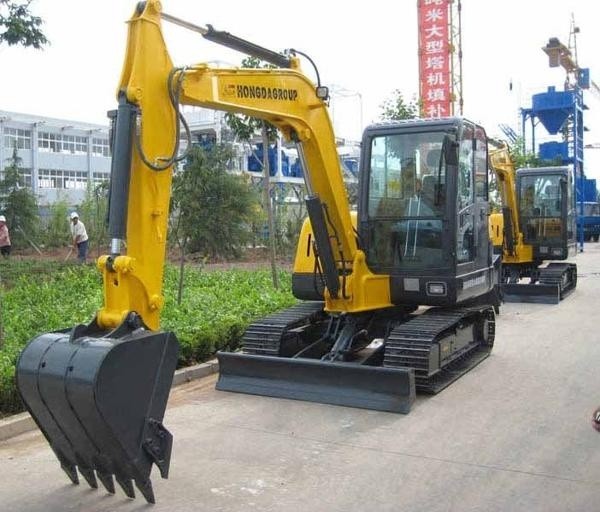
[
  {"xmin": 541, "ymin": 184, "xmax": 560, "ymax": 210},
  {"xmin": 422, "ymin": 148, "xmax": 447, "ymax": 200}
]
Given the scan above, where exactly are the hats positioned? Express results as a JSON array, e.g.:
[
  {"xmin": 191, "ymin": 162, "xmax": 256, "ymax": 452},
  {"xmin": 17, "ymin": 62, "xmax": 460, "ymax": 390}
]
[
  {"xmin": 70, "ymin": 211, "xmax": 79, "ymax": 219},
  {"xmin": 0, "ymin": 215, "xmax": 6, "ymax": 222}
]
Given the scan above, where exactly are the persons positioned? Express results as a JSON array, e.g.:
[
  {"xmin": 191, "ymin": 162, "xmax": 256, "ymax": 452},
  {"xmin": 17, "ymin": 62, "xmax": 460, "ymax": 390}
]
[
  {"xmin": 0, "ymin": 215, "xmax": 12, "ymax": 258},
  {"xmin": 69, "ymin": 212, "xmax": 89, "ymax": 263}
]
